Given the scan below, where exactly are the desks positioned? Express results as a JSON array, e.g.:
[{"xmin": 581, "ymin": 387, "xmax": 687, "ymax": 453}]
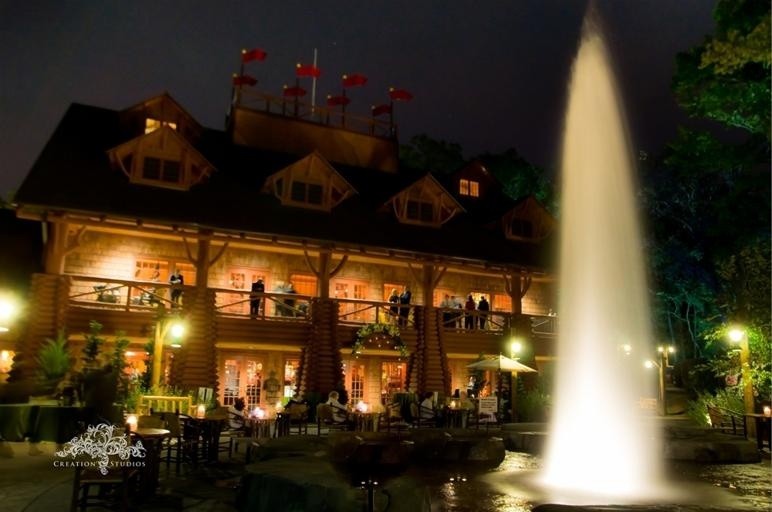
[{"xmin": 743, "ymin": 411, "xmax": 771, "ymax": 456}]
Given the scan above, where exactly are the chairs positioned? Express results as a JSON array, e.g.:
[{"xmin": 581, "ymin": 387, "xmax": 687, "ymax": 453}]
[
  {"xmin": 62, "ymin": 380, "xmax": 508, "ymax": 511},
  {"xmin": 705, "ymin": 402, "xmax": 747, "ymax": 440},
  {"xmin": 93, "ymin": 284, "xmax": 165, "ymax": 310}
]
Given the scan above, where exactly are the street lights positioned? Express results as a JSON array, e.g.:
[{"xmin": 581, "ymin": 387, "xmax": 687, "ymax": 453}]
[
  {"xmin": 724, "ymin": 322, "xmax": 757, "ymax": 436},
  {"xmin": 511, "ymin": 340, "xmax": 523, "ymax": 423},
  {"xmin": 150, "ymin": 316, "xmax": 190, "ymax": 389},
  {"xmin": 624, "ymin": 344, "xmax": 678, "ymax": 416}
]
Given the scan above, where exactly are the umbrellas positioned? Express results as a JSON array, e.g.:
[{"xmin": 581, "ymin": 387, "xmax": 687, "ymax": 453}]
[{"xmin": 462, "ymin": 352, "xmax": 539, "ymax": 419}]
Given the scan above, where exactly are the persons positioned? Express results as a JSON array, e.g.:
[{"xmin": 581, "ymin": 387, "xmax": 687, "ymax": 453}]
[
  {"xmin": 325, "ymin": 390, "xmax": 355, "ymax": 432},
  {"xmin": 168, "ymin": 269, "xmax": 183, "ymax": 309},
  {"xmin": 227, "ymin": 399, "xmax": 253, "ymax": 437},
  {"xmin": 398, "ymin": 286, "xmax": 411, "ymax": 326},
  {"xmin": 274, "ymin": 281, "xmax": 287, "ymax": 316},
  {"xmin": 284, "ymin": 283, "xmax": 296, "ymax": 317},
  {"xmin": 388, "ymin": 289, "xmax": 399, "ymax": 321},
  {"xmin": 250, "ymin": 279, "xmax": 264, "ymax": 320},
  {"xmin": 440, "ymin": 295, "xmax": 489, "ymax": 329},
  {"xmin": 55, "ymin": 358, "xmax": 116, "ymax": 406},
  {"xmin": 419, "ymin": 392, "xmax": 440, "ymax": 423}
]
